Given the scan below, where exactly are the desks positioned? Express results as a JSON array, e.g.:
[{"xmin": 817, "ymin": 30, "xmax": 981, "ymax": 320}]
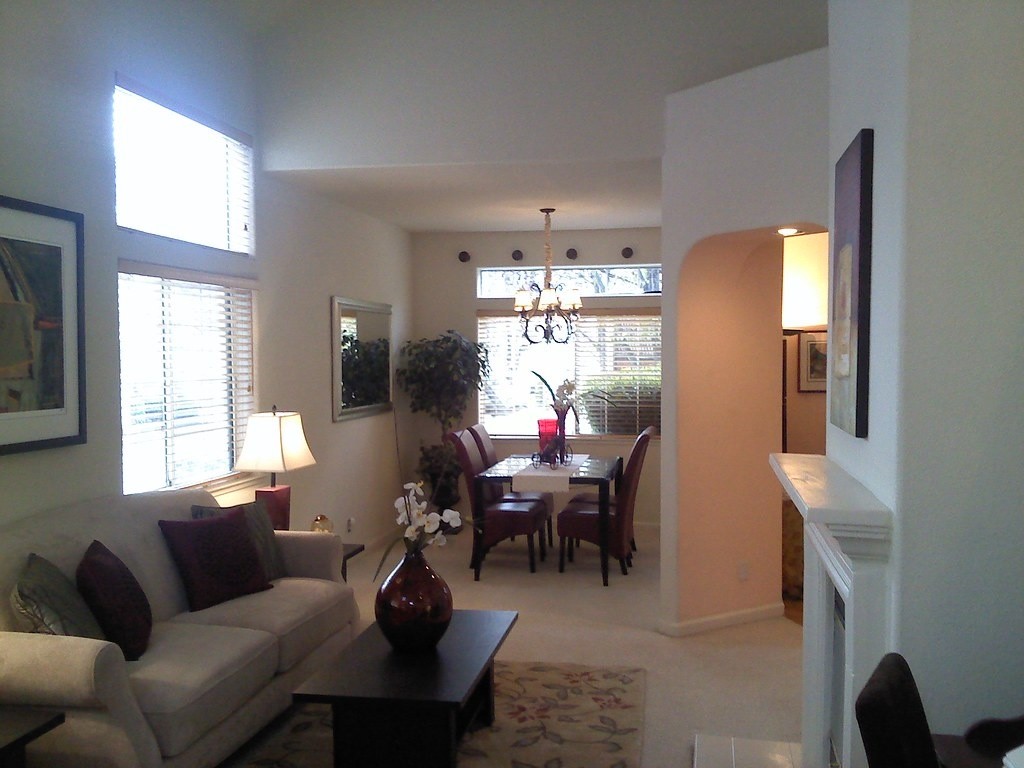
[{"xmin": 291, "ymin": 605, "xmax": 519, "ymax": 768}]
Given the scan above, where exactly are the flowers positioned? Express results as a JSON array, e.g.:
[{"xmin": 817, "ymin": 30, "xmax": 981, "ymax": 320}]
[
  {"xmin": 531, "ymin": 370, "xmax": 618, "ymax": 424},
  {"xmin": 370, "ymin": 405, "xmax": 465, "ymax": 582}
]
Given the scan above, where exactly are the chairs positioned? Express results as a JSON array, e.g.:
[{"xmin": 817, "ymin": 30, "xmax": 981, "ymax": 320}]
[
  {"xmin": 448, "ymin": 424, "xmax": 655, "ymax": 576},
  {"xmin": 854, "ymin": 652, "xmax": 937, "ymax": 768}
]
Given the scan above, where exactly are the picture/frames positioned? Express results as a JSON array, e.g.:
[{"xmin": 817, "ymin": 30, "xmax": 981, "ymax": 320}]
[
  {"xmin": 798, "ymin": 331, "xmax": 827, "ymax": 393},
  {"xmin": 0, "ymin": 192, "xmax": 88, "ymax": 457}
]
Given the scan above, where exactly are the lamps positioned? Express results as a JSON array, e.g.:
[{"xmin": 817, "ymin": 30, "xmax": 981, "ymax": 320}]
[
  {"xmin": 232, "ymin": 404, "xmax": 317, "ymax": 530},
  {"xmin": 514, "ymin": 206, "xmax": 583, "ymax": 343}
]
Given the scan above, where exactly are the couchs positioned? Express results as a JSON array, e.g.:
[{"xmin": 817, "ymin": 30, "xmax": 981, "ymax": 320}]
[{"xmin": 0, "ymin": 486, "xmax": 361, "ymax": 768}]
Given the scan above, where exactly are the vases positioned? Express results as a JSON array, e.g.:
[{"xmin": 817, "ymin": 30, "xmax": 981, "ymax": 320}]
[
  {"xmin": 375, "ymin": 542, "xmax": 452, "ymax": 660},
  {"xmin": 552, "ymin": 406, "xmax": 570, "ymax": 465}
]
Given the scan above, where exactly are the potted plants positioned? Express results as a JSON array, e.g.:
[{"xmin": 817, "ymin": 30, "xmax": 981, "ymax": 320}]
[{"xmin": 394, "ymin": 329, "xmax": 490, "ymax": 536}]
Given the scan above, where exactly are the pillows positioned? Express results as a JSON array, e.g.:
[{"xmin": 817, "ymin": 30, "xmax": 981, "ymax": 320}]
[
  {"xmin": 75, "ymin": 539, "xmax": 152, "ymax": 660},
  {"xmin": 191, "ymin": 499, "xmax": 286, "ymax": 581},
  {"xmin": 158, "ymin": 506, "xmax": 275, "ymax": 614},
  {"xmin": 9, "ymin": 554, "xmax": 105, "ymax": 645}
]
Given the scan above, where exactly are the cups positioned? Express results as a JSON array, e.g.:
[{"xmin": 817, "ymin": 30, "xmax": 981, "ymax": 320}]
[{"xmin": 538, "ymin": 419, "xmax": 559, "ymax": 458}]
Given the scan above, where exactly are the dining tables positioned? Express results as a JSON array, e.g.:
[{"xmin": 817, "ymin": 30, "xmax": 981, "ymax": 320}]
[{"xmin": 470, "ymin": 454, "xmax": 623, "ymax": 588}]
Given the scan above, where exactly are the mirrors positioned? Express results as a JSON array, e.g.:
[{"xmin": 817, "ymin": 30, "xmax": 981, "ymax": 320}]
[{"xmin": 331, "ymin": 296, "xmax": 393, "ymax": 421}]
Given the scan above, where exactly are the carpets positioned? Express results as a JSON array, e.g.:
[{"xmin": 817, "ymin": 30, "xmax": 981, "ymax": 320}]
[{"xmin": 233, "ymin": 660, "xmax": 646, "ymax": 768}]
